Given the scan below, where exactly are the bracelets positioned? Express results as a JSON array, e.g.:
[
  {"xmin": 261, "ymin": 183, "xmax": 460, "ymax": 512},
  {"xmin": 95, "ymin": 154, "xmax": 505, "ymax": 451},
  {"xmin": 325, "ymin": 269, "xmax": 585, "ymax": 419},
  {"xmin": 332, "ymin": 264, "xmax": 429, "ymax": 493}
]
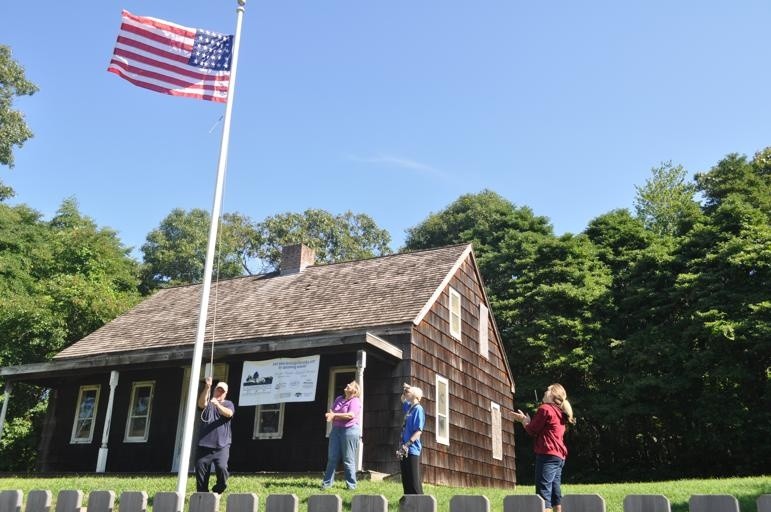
[{"xmin": 407, "ymin": 439, "xmax": 413, "ymax": 446}]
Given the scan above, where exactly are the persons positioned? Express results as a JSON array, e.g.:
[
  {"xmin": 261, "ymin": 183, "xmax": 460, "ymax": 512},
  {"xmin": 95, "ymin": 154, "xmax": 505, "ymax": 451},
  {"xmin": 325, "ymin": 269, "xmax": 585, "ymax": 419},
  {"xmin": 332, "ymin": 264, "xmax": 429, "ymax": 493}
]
[
  {"xmin": 319, "ymin": 381, "xmax": 362, "ymax": 492},
  {"xmin": 395, "ymin": 381, "xmax": 426, "ymax": 493},
  {"xmin": 507, "ymin": 382, "xmax": 577, "ymax": 512},
  {"xmin": 196, "ymin": 377, "xmax": 235, "ymax": 499}
]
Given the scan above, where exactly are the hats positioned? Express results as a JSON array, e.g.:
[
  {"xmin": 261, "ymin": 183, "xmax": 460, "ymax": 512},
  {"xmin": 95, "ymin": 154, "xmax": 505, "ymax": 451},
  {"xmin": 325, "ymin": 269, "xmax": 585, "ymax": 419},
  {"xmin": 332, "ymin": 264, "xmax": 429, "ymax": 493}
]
[{"xmin": 215, "ymin": 381, "xmax": 228, "ymax": 393}]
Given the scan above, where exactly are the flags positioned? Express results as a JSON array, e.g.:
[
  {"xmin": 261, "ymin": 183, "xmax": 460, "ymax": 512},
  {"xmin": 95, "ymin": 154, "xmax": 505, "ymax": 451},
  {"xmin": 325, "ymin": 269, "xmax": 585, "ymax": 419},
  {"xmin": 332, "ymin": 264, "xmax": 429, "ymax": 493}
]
[{"xmin": 106, "ymin": 9, "xmax": 234, "ymax": 103}]
